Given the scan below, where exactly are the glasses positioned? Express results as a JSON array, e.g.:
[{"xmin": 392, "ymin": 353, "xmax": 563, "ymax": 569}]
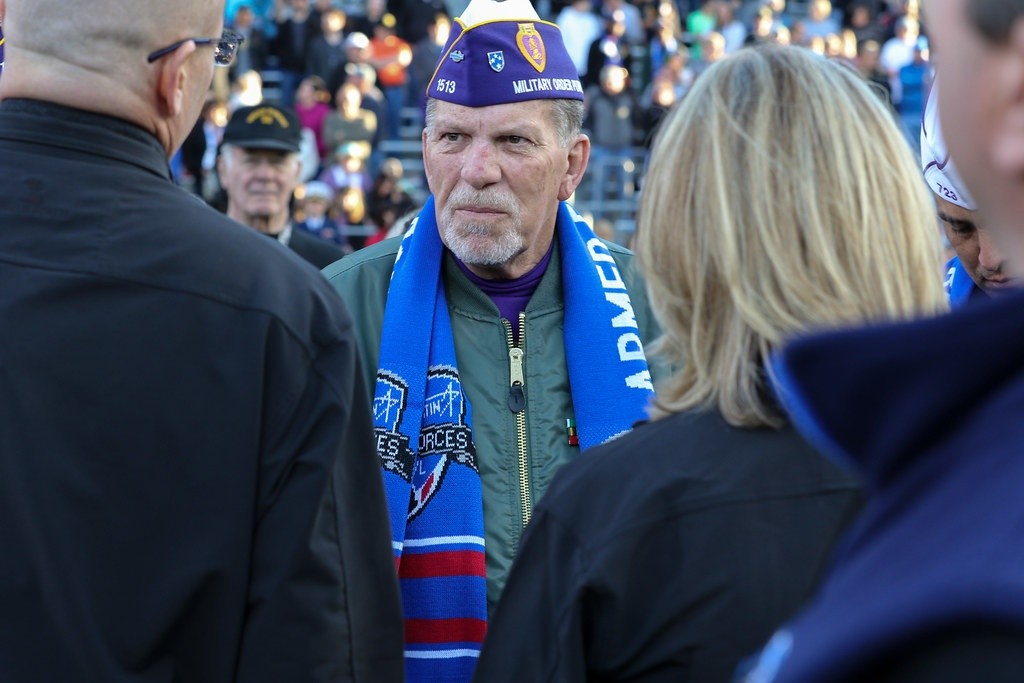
[{"xmin": 148, "ymin": 27, "xmax": 244, "ymax": 68}]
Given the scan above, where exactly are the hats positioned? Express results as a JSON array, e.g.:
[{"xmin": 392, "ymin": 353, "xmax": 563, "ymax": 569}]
[
  {"xmin": 223, "ymin": 105, "xmax": 302, "ymax": 153},
  {"xmin": 426, "ymin": 0, "xmax": 585, "ymax": 106},
  {"xmin": 920, "ymin": 71, "xmax": 979, "ymax": 212}
]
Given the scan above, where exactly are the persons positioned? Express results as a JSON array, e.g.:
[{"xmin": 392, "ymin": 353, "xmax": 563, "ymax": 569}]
[{"xmin": 0, "ymin": 0, "xmax": 1024, "ymax": 683}]
